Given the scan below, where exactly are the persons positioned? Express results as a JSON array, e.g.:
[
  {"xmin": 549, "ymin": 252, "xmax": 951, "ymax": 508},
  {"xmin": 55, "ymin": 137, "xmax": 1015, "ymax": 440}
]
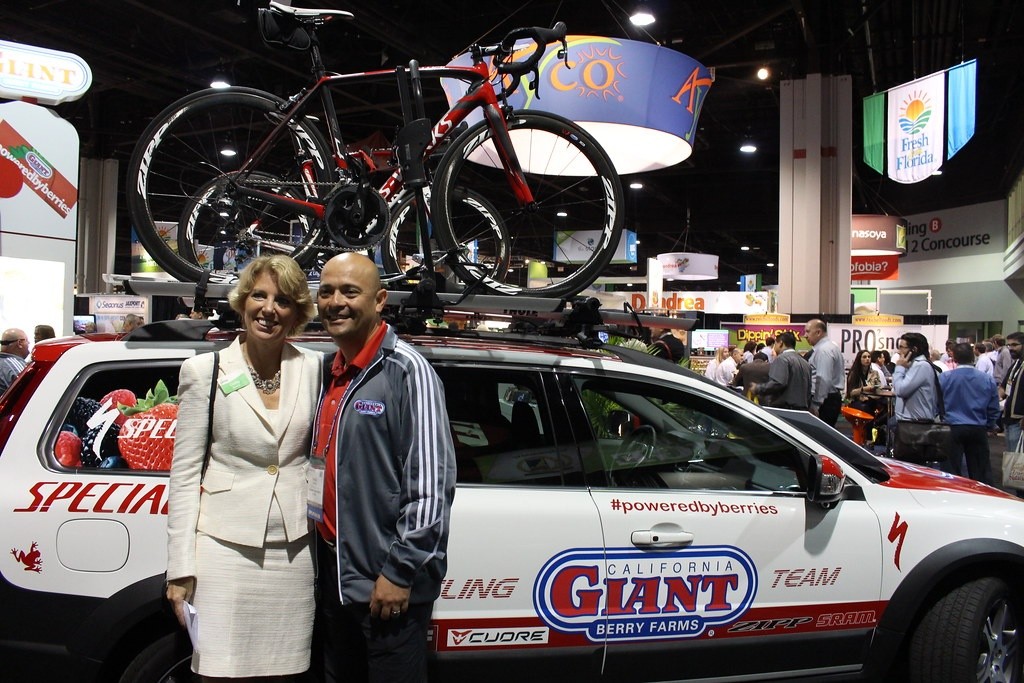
[
  {"xmin": 34, "ymin": 325, "xmax": 55, "ymax": 344},
  {"xmin": 727, "ymin": 352, "xmax": 771, "ymax": 406},
  {"xmin": 309, "ymin": 252, "xmax": 456, "ymax": 683},
  {"xmin": 175, "ymin": 308, "xmax": 220, "ymax": 332},
  {"xmin": 0, "ymin": 328, "xmax": 31, "ymax": 396},
  {"xmin": 704, "ymin": 346, "xmax": 729, "ymax": 381},
  {"xmin": 715, "ymin": 349, "xmax": 743, "ymax": 387},
  {"xmin": 165, "ymin": 254, "xmax": 324, "ymax": 683},
  {"xmin": 122, "ymin": 314, "xmax": 144, "ymax": 334},
  {"xmin": 749, "ymin": 332, "xmax": 812, "ymax": 409},
  {"xmin": 84, "ymin": 322, "xmax": 96, "ymax": 333},
  {"xmin": 802, "ymin": 319, "xmax": 844, "ymax": 428},
  {"xmin": 891, "ymin": 332, "xmax": 1024, "ymax": 499},
  {"xmin": 743, "ymin": 337, "xmax": 777, "ymax": 363},
  {"xmin": 845, "ymin": 350, "xmax": 895, "ymax": 448}
]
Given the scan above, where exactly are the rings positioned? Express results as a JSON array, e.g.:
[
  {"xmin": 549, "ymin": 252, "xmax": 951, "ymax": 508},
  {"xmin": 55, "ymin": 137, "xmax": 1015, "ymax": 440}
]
[{"xmin": 392, "ymin": 609, "xmax": 401, "ymax": 615}]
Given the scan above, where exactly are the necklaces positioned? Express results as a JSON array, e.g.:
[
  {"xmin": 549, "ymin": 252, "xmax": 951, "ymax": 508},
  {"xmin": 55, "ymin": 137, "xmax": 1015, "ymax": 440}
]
[{"xmin": 242, "ymin": 344, "xmax": 280, "ymax": 395}]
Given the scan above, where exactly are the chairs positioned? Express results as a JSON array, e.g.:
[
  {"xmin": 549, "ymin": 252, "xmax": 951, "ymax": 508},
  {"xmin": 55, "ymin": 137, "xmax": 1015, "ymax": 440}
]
[{"xmin": 508, "ymin": 400, "xmax": 541, "ymax": 470}]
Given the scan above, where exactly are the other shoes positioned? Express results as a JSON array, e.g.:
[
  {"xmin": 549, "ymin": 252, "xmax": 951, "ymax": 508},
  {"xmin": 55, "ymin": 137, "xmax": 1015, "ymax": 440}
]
[{"xmin": 987, "ymin": 424, "xmax": 999, "ymax": 437}]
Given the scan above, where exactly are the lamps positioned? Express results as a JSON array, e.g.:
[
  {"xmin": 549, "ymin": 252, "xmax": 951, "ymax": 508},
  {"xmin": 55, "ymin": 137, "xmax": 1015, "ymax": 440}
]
[
  {"xmin": 655, "ymin": 204, "xmax": 720, "ymax": 282},
  {"xmin": 850, "ymin": 156, "xmax": 908, "ymax": 256},
  {"xmin": 440, "ymin": 0, "xmax": 711, "ymax": 176}
]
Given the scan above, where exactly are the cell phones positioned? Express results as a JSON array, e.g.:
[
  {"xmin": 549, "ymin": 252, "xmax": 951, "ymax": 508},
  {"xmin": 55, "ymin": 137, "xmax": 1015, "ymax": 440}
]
[{"xmin": 904, "ymin": 349, "xmax": 916, "ymax": 361}]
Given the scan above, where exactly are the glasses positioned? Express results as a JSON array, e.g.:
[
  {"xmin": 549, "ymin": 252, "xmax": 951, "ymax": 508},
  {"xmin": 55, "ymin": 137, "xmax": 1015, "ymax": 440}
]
[
  {"xmin": 898, "ymin": 345, "xmax": 912, "ymax": 353},
  {"xmin": 1006, "ymin": 342, "xmax": 1023, "ymax": 349}
]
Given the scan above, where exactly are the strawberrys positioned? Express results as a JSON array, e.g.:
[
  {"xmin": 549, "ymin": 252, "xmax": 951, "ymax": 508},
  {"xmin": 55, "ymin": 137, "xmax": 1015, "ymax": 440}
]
[{"xmin": 117, "ymin": 379, "xmax": 176, "ymax": 472}]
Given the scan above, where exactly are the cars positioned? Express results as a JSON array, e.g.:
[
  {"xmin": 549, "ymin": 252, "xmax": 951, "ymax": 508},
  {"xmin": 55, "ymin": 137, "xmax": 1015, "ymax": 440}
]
[{"xmin": 0, "ymin": 307, "xmax": 1023, "ymax": 682}]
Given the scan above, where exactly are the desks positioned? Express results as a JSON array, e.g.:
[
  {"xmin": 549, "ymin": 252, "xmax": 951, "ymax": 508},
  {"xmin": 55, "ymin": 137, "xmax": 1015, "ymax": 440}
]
[{"xmin": 863, "ymin": 390, "xmax": 895, "ymax": 457}]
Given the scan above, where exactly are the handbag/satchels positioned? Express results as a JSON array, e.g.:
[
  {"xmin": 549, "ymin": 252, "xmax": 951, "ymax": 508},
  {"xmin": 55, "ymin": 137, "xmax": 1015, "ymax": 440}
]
[
  {"xmin": 1001, "ymin": 430, "xmax": 1024, "ymax": 492},
  {"xmin": 893, "ymin": 418, "xmax": 952, "ymax": 465}
]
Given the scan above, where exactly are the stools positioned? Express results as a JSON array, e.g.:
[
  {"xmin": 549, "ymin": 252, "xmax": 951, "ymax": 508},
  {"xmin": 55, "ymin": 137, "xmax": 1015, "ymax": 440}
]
[{"xmin": 840, "ymin": 407, "xmax": 875, "ymax": 448}]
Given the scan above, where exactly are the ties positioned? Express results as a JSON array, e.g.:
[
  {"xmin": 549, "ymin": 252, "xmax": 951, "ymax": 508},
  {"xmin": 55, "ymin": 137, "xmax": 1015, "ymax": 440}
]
[{"xmin": 803, "ymin": 349, "xmax": 814, "ymax": 362}]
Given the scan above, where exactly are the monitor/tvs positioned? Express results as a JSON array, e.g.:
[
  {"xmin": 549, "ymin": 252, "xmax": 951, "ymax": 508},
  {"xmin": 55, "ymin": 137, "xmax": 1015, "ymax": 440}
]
[
  {"xmin": 692, "ymin": 328, "xmax": 729, "ymax": 351},
  {"xmin": 73, "ymin": 314, "xmax": 96, "ymax": 333}
]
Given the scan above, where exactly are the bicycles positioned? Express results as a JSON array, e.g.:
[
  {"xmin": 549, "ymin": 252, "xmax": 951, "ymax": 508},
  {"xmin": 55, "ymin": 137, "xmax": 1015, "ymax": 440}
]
[
  {"xmin": 121, "ymin": 1, "xmax": 623, "ymax": 333},
  {"xmin": 178, "ymin": 107, "xmax": 511, "ymax": 305}
]
